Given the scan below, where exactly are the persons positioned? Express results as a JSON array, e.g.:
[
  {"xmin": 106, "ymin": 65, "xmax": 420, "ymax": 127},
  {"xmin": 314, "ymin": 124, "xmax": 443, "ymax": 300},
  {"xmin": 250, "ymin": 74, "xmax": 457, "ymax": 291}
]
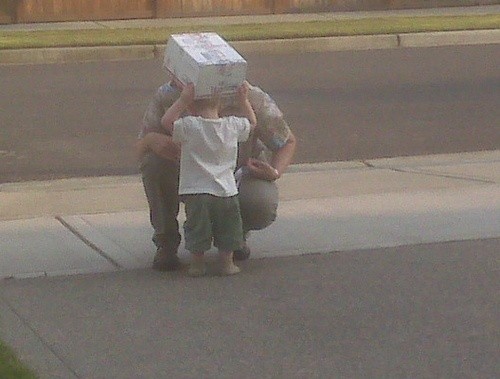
[
  {"xmin": 161, "ymin": 80, "xmax": 258, "ymax": 276},
  {"xmin": 132, "ymin": 74, "xmax": 298, "ymax": 270}
]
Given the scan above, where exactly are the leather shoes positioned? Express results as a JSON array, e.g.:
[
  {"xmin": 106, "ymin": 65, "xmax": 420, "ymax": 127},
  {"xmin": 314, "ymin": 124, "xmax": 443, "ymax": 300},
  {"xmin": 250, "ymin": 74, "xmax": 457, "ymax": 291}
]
[
  {"xmin": 153, "ymin": 246, "xmax": 179, "ymax": 272},
  {"xmin": 232, "ymin": 234, "xmax": 250, "ymax": 260}
]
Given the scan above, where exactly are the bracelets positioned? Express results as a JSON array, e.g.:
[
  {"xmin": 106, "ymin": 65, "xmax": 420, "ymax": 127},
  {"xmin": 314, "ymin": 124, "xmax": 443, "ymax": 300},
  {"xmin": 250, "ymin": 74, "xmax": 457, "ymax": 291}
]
[{"xmin": 275, "ymin": 167, "xmax": 280, "ymax": 179}]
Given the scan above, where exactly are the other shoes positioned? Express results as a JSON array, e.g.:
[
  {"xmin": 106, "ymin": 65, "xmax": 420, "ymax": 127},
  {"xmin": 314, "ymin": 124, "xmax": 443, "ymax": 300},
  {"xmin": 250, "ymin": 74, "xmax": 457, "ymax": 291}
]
[{"xmin": 188, "ymin": 255, "xmax": 240, "ymax": 276}]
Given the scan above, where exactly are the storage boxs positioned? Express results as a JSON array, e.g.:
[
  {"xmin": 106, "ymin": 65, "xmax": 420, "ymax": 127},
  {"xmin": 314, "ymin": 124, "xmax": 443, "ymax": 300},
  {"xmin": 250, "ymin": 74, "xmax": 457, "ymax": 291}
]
[{"xmin": 161, "ymin": 32, "xmax": 248, "ymax": 101}]
